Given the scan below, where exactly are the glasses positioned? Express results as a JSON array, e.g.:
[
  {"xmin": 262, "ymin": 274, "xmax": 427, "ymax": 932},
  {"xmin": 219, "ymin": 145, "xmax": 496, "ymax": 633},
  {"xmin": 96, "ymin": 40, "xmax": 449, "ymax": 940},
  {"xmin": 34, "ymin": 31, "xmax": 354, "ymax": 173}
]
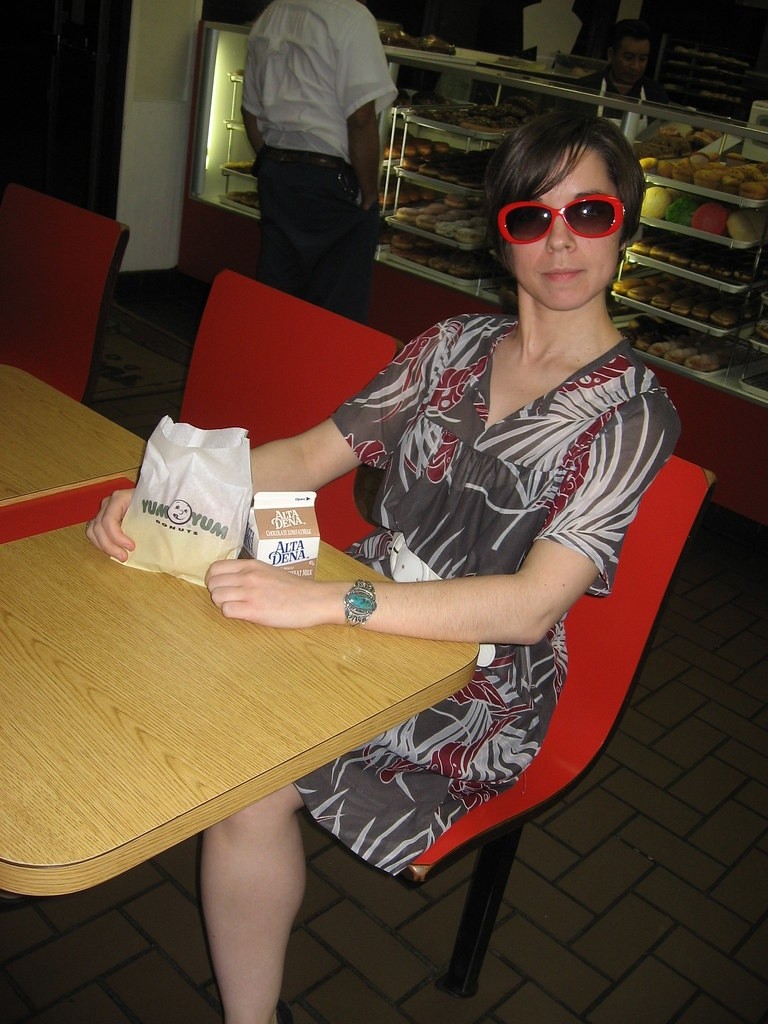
[{"xmin": 495, "ymin": 194, "xmax": 626, "ymax": 245}]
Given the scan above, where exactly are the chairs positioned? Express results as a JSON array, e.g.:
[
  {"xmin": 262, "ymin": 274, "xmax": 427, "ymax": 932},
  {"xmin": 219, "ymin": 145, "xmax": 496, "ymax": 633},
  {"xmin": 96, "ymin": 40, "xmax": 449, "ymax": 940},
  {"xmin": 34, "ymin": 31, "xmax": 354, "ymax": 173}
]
[
  {"xmin": 180, "ymin": 269, "xmax": 407, "ymax": 549},
  {"xmin": 400, "ymin": 457, "xmax": 716, "ymax": 1001},
  {"xmin": 0, "ymin": 185, "xmax": 130, "ymax": 404}
]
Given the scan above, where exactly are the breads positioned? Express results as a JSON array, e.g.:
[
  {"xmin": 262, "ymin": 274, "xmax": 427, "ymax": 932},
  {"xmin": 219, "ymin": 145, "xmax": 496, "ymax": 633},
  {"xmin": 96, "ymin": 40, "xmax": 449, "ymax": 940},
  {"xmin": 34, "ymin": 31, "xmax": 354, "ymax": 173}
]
[
  {"xmin": 663, "ymin": 45, "xmax": 750, "ymax": 102},
  {"xmin": 223, "ymin": 159, "xmax": 260, "ymax": 210},
  {"xmin": 380, "ymin": 29, "xmax": 557, "ymax": 307},
  {"xmin": 604, "ymin": 125, "xmax": 768, "ymax": 373}
]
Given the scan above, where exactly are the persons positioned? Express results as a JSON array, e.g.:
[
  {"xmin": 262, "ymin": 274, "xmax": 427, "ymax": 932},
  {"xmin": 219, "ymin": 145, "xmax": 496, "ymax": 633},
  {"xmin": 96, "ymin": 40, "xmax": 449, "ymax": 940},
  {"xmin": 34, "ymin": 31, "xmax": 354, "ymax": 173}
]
[
  {"xmin": 85, "ymin": 106, "xmax": 683, "ymax": 1023},
  {"xmin": 577, "ymin": 19, "xmax": 670, "ymax": 102},
  {"xmin": 240, "ymin": 1, "xmax": 399, "ymax": 322}
]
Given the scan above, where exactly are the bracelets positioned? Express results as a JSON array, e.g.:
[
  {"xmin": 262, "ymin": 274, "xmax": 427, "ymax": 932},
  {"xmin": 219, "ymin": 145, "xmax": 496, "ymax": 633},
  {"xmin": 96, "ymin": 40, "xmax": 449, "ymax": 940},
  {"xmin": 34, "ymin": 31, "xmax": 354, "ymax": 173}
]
[{"xmin": 342, "ymin": 580, "xmax": 378, "ymax": 630}]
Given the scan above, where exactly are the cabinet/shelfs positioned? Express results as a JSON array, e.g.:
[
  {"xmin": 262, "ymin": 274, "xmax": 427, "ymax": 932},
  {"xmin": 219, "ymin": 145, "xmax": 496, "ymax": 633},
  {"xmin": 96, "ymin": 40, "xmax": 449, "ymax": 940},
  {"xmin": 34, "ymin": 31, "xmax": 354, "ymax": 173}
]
[{"xmin": 170, "ymin": 19, "xmax": 768, "ymax": 532}]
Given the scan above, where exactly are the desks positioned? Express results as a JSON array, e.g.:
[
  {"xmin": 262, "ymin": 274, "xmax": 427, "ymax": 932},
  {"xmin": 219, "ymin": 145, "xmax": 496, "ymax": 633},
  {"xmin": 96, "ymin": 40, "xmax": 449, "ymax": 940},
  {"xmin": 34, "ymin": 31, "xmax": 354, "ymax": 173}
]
[{"xmin": 0, "ymin": 367, "xmax": 479, "ymax": 897}]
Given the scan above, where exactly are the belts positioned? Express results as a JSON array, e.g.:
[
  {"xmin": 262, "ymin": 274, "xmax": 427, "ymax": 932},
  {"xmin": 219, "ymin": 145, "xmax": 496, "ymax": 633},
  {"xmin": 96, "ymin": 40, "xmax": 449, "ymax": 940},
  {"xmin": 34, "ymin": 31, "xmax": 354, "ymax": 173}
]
[{"xmin": 258, "ymin": 146, "xmax": 347, "ymax": 172}]
